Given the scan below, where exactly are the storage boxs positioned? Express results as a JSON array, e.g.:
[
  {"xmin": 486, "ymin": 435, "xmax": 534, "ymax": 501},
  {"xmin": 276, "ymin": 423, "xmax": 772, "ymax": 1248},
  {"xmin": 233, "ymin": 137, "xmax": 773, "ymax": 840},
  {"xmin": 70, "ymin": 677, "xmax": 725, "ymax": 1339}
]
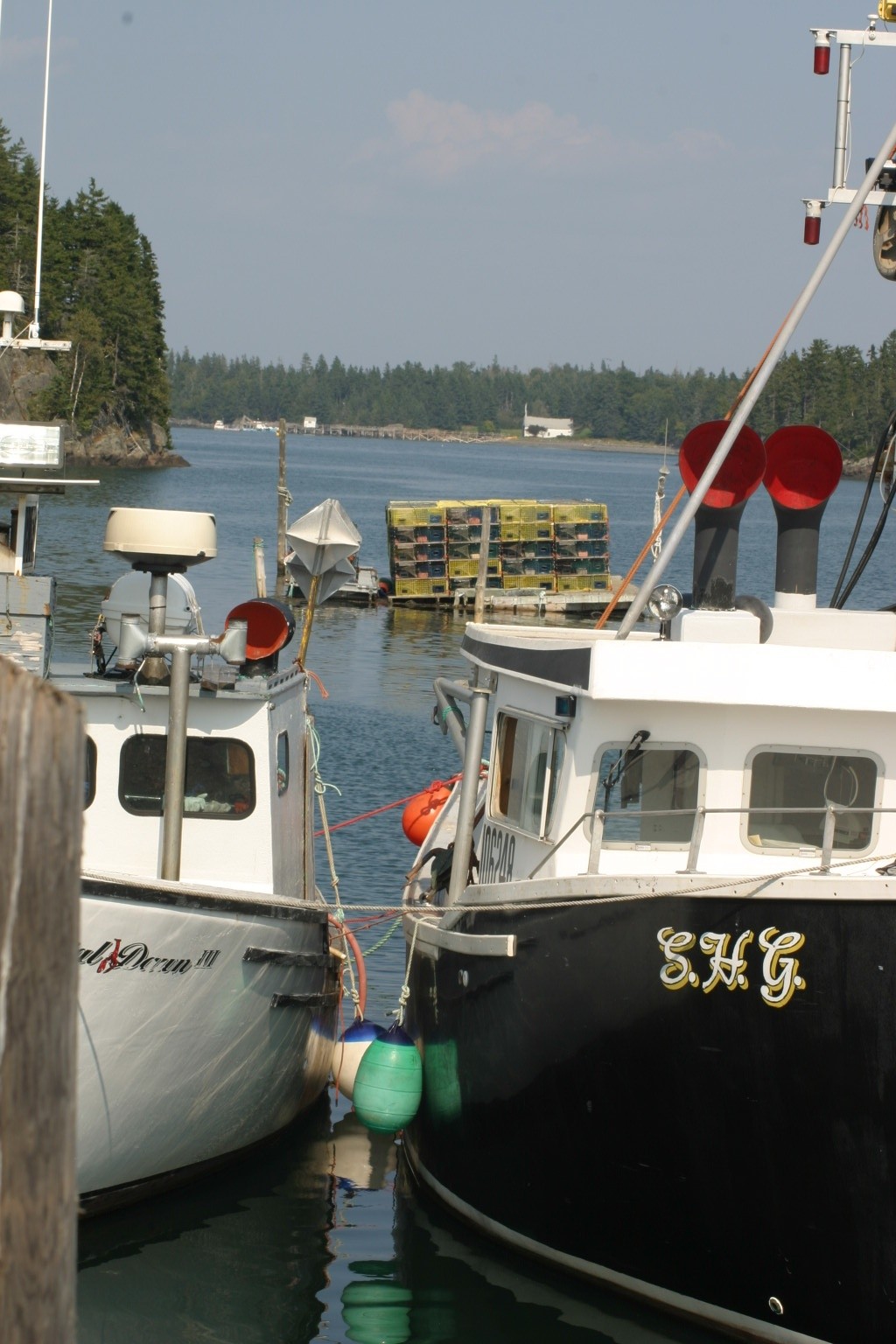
[
  {"xmin": 386, "ymin": 498, "xmax": 609, "ymax": 596},
  {"xmin": 0, "ymin": 576, "xmax": 55, "ymax": 679}
]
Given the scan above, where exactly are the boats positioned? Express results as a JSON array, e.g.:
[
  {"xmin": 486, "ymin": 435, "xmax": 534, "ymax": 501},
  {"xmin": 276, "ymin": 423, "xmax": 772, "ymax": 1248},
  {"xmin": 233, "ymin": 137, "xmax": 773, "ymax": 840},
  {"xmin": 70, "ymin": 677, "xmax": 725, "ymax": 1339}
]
[
  {"xmin": 382, "ymin": 498, "xmax": 641, "ymax": 611},
  {"xmin": 1, "ymin": 0, "xmax": 349, "ymax": 1216},
  {"xmin": 214, "ymin": 419, "xmax": 280, "ymax": 433},
  {"xmin": 294, "ymin": 1, "xmax": 896, "ymax": 1344},
  {"xmin": 282, "ymin": 498, "xmax": 379, "ymax": 609}
]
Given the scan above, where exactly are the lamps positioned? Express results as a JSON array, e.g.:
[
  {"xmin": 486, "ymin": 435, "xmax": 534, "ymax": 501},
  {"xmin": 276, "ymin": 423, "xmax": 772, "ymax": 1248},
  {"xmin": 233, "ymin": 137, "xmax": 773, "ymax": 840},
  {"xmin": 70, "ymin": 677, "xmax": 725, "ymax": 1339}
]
[
  {"xmin": 649, "ymin": 585, "xmax": 684, "ymax": 640},
  {"xmin": 0, "ymin": 419, "xmax": 64, "ymax": 470}
]
